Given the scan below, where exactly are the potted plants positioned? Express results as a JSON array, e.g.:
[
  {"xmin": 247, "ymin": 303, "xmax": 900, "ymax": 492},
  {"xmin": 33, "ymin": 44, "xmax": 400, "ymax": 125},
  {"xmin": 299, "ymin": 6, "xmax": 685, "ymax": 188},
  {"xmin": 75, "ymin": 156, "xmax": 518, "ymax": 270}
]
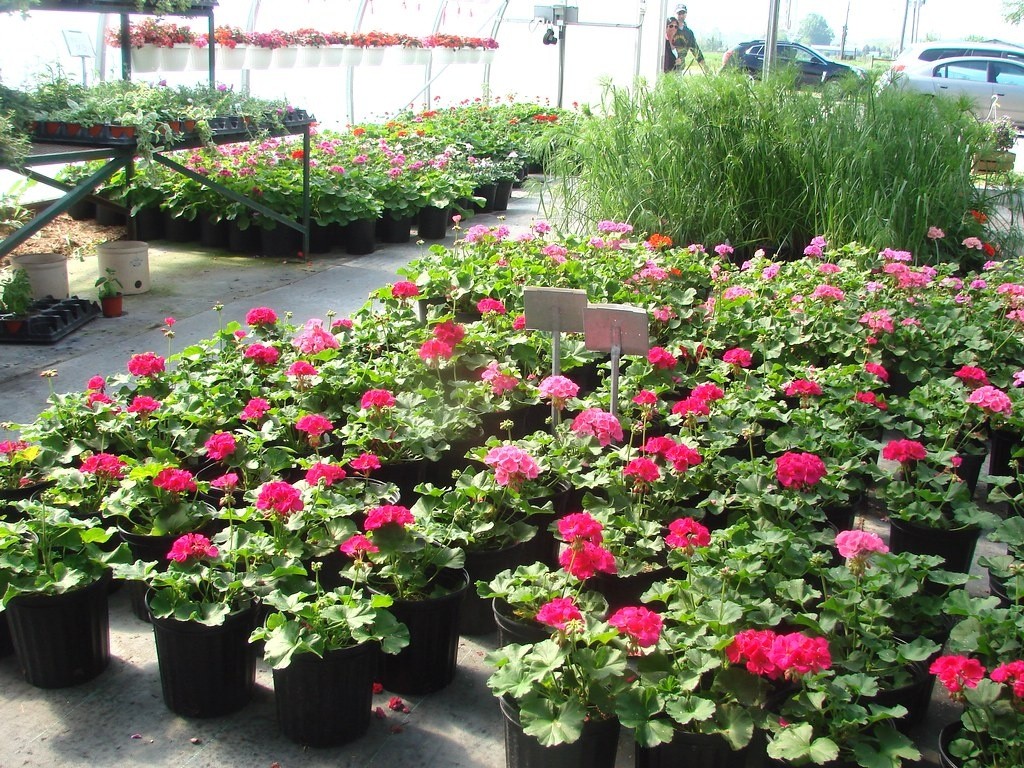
[{"xmin": 0, "ymin": 13, "xmax": 1024, "ymax": 766}]
